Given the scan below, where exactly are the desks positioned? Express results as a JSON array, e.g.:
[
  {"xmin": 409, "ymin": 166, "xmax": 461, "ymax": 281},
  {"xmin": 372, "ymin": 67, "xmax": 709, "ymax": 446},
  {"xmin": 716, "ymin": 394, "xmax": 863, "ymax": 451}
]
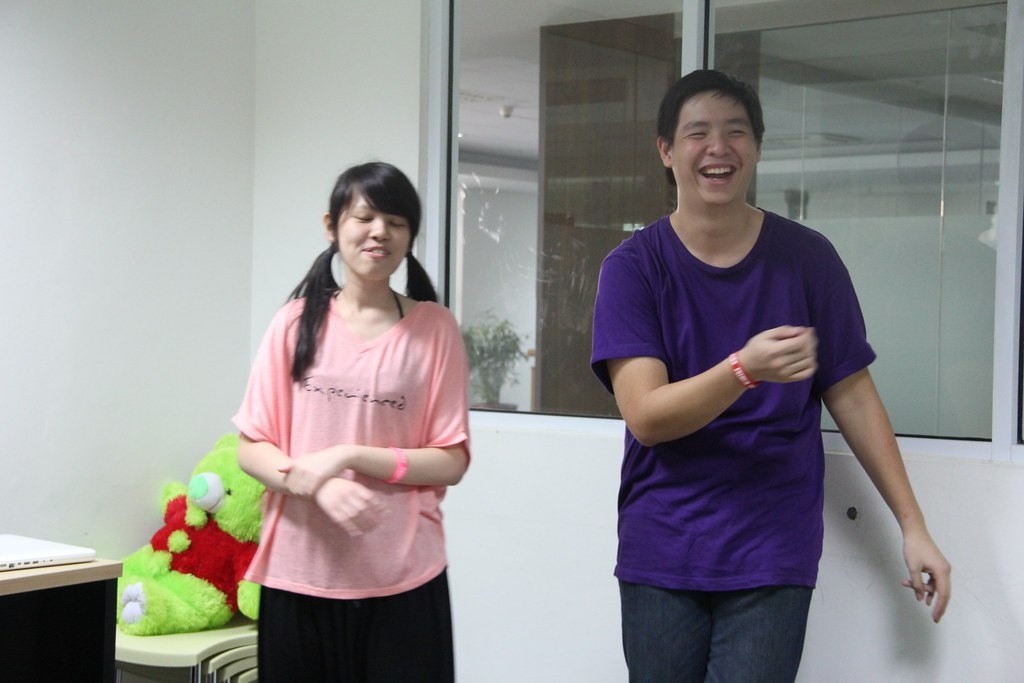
[{"xmin": 0, "ymin": 555, "xmax": 123, "ymax": 683}]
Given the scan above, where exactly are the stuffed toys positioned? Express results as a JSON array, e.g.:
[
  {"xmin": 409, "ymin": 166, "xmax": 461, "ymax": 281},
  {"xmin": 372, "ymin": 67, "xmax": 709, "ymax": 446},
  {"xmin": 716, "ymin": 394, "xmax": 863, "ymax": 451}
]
[{"xmin": 111, "ymin": 432, "xmax": 264, "ymax": 635}]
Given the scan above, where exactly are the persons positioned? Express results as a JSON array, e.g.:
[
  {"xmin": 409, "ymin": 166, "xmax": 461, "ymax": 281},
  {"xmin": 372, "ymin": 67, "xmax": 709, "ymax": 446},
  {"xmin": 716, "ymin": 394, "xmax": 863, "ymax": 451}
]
[
  {"xmin": 229, "ymin": 161, "xmax": 473, "ymax": 683},
  {"xmin": 590, "ymin": 68, "xmax": 951, "ymax": 683}
]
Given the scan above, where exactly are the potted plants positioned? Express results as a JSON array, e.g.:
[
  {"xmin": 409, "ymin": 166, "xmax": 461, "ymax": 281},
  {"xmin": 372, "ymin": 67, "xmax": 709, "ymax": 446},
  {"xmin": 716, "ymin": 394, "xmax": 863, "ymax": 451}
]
[{"xmin": 461, "ymin": 311, "xmax": 531, "ymax": 413}]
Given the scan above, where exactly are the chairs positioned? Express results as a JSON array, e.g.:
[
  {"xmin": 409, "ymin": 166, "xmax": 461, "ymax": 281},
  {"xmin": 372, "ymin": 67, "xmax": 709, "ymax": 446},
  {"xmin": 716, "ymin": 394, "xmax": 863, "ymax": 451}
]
[{"xmin": 115, "ymin": 611, "xmax": 259, "ymax": 682}]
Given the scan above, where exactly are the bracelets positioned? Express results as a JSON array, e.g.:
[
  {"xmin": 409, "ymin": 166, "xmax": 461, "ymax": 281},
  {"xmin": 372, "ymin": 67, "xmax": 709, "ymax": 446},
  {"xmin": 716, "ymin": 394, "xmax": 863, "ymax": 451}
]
[
  {"xmin": 386, "ymin": 444, "xmax": 408, "ymax": 485},
  {"xmin": 727, "ymin": 351, "xmax": 760, "ymax": 390}
]
